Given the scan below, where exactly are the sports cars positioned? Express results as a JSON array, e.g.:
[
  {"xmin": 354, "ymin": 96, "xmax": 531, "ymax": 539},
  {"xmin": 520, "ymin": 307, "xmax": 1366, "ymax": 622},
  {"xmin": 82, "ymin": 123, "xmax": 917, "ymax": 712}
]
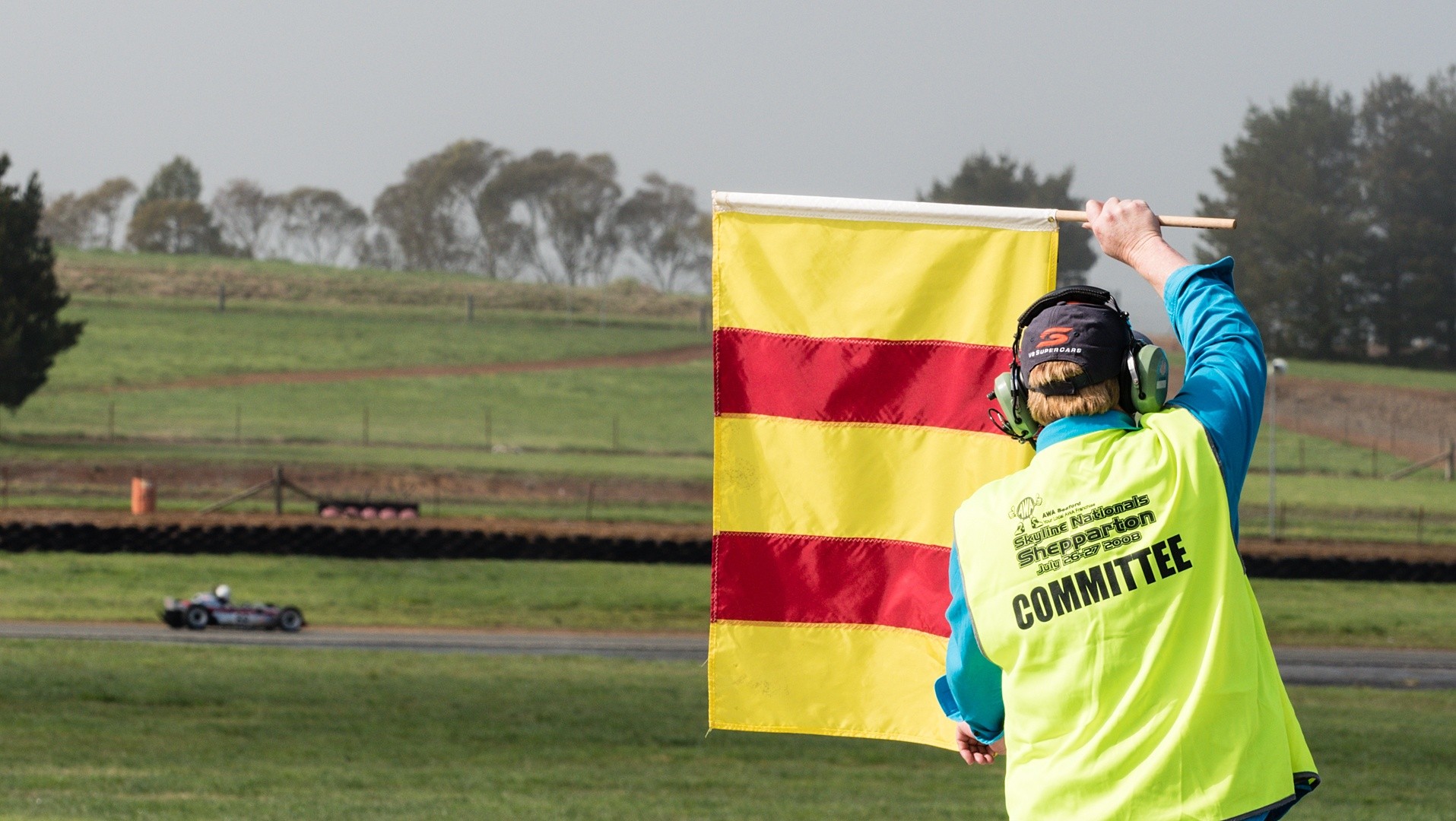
[{"xmin": 159, "ymin": 593, "xmax": 314, "ymax": 635}]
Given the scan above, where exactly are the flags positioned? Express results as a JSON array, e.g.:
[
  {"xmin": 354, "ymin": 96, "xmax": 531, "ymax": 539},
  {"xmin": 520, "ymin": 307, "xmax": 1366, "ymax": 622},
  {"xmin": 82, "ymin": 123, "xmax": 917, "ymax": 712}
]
[{"xmin": 707, "ymin": 193, "xmax": 1058, "ymax": 751}]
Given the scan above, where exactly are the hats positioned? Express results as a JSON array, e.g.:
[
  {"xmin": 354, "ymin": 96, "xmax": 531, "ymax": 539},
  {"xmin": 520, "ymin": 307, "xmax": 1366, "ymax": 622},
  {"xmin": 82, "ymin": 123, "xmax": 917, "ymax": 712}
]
[{"xmin": 1018, "ymin": 302, "xmax": 1126, "ymax": 395}]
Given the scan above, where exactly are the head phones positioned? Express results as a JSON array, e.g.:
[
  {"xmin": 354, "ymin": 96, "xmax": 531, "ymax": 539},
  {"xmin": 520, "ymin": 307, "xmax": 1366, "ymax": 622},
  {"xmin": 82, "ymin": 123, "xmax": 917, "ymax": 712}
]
[{"xmin": 994, "ymin": 285, "xmax": 1172, "ymax": 443}]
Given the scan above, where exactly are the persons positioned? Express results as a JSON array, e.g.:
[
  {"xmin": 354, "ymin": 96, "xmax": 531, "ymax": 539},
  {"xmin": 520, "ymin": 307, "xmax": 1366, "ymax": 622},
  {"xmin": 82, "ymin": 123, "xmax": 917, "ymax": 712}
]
[
  {"xmin": 944, "ymin": 196, "xmax": 1321, "ymax": 821},
  {"xmin": 216, "ymin": 585, "xmax": 233, "ymax": 609}
]
